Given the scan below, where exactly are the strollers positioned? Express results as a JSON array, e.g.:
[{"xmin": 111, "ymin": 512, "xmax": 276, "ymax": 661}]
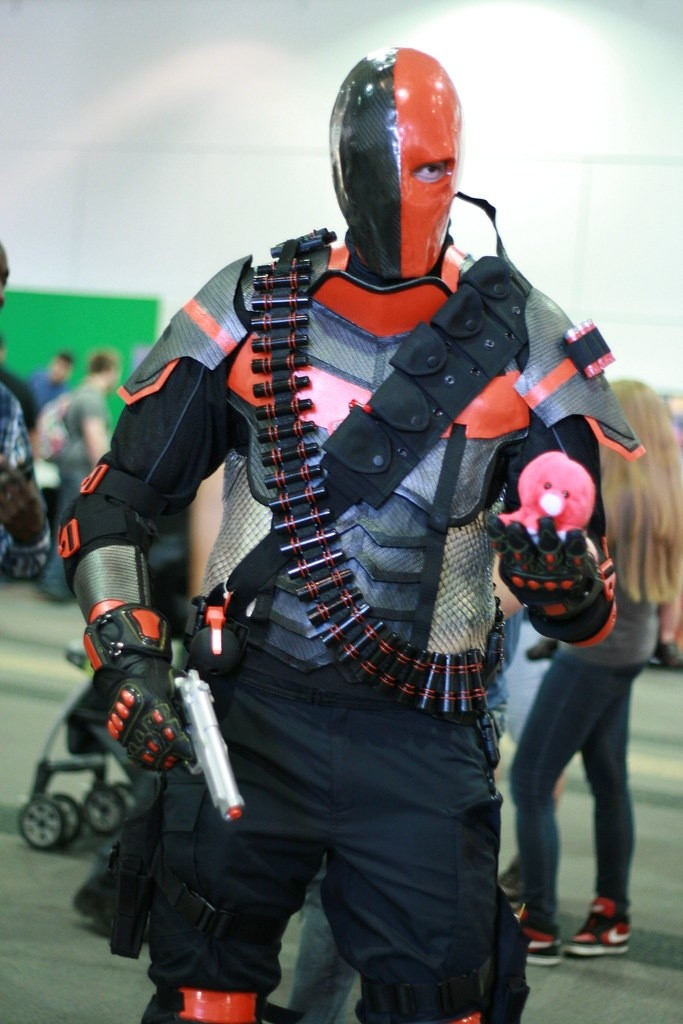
[{"xmin": 16, "ymin": 642, "xmax": 134, "ymax": 855}]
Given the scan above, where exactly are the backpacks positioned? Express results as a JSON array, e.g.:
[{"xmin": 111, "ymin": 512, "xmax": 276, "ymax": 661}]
[{"xmin": 34, "ymin": 391, "xmax": 72, "ymax": 461}]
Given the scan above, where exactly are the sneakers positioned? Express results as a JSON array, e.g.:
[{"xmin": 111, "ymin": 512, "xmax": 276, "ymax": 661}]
[
  {"xmin": 564, "ymin": 895, "xmax": 633, "ymax": 958},
  {"xmin": 514, "ymin": 904, "xmax": 561, "ymax": 967}
]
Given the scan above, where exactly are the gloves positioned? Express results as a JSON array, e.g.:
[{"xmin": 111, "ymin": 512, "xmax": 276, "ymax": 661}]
[
  {"xmin": 83, "ymin": 603, "xmax": 198, "ymax": 773},
  {"xmin": 480, "ymin": 512, "xmax": 618, "ymax": 649}
]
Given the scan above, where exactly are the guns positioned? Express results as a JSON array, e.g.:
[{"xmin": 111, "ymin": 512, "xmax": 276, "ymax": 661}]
[{"xmin": 173, "ymin": 665, "xmax": 245, "ymax": 826}]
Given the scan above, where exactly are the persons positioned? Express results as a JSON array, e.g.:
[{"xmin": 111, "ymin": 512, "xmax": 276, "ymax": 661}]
[
  {"xmin": 0, "ymin": 245, "xmax": 49, "ymax": 590},
  {"xmin": 28, "ymin": 47, "xmax": 683, "ymax": 1023}
]
